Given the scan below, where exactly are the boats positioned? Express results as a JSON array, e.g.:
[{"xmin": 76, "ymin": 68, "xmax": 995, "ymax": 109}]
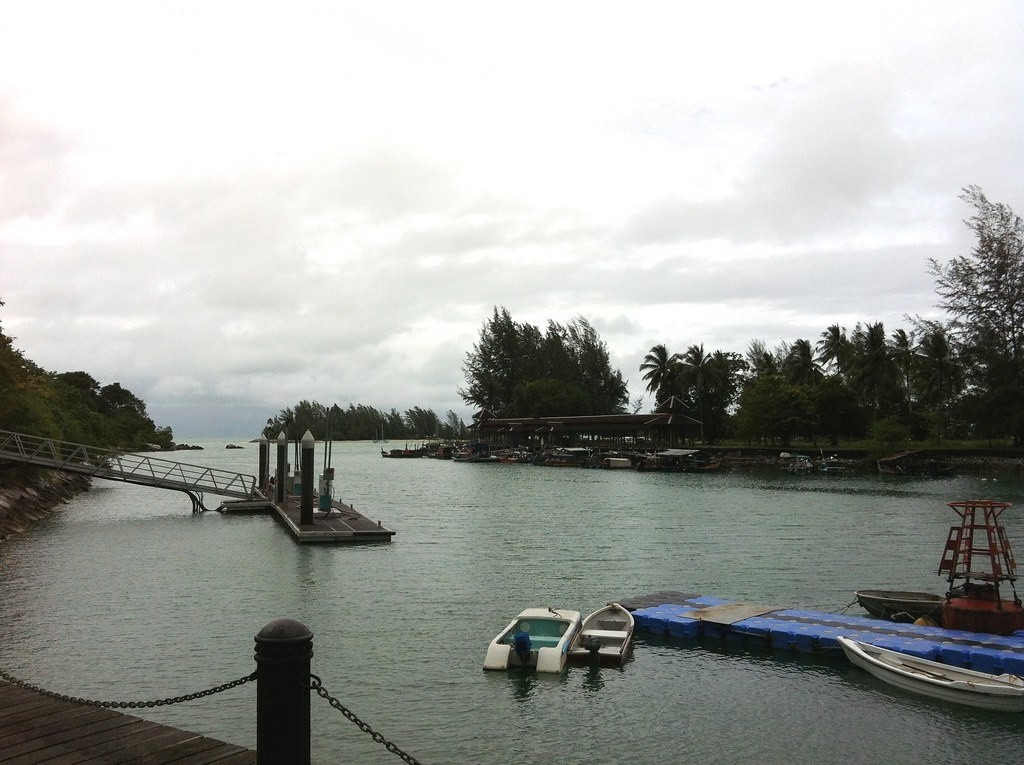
[
  {"xmin": 836, "ymin": 635, "xmax": 1024, "ymax": 713},
  {"xmin": 853, "ymin": 589, "xmax": 945, "ymax": 627},
  {"xmin": 565, "ymin": 601, "xmax": 635, "ymax": 670},
  {"xmin": 373, "ymin": 432, "xmax": 964, "ymax": 478},
  {"xmin": 482, "ymin": 607, "xmax": 581, "ymax": 674}
]
